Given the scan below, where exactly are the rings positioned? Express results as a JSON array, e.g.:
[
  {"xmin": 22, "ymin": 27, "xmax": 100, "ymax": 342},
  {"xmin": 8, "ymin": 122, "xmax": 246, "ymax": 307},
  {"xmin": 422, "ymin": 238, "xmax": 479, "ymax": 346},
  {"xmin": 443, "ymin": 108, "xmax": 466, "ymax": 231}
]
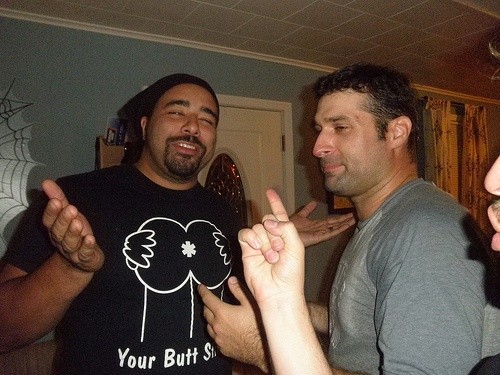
[{"xmin": 329, "ymin": 227, "xmax": 335, "ymax": 232}]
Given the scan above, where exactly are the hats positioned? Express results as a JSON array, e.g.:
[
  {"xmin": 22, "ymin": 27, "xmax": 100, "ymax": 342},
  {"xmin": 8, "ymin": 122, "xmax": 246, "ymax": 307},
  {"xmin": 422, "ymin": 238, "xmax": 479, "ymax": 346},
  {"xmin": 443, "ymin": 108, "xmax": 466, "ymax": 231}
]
[{"xmin": 149, "ymin": 73, "xmax": 220, "ymax": 121}]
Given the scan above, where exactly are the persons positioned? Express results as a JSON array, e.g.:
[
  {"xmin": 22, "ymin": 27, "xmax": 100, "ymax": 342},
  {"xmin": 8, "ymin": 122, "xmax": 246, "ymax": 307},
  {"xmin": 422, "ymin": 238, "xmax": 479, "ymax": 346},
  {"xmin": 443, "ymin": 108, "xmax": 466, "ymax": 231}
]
[
  {"xmin": 239, "ymin": 153, "xmax": 500, "ymax": 375},
  {"xmin": 197, "ymin": 62, "xmax": 500, "ymax": 375},
  {"xmin": 0, "ymin": 74, "xmax": 357, "ymax": 375}
]
